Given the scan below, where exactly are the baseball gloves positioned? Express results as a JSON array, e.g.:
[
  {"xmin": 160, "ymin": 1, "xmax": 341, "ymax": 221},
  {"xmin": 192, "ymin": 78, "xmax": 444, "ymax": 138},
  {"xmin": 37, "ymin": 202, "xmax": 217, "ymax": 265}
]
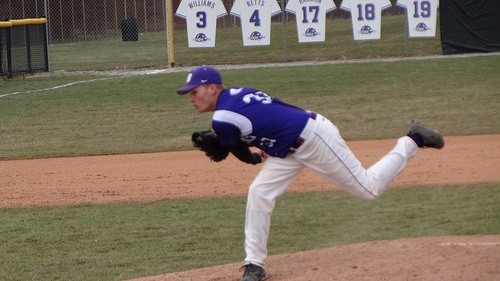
[{"xmin": 193, "ymin": 129, "xmax": 230, "ymax": 163}]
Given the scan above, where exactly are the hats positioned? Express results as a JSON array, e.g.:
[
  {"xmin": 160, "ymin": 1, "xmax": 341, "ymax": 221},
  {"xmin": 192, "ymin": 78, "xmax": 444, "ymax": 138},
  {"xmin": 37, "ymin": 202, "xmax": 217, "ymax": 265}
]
[{"xmin": 177, "ymin": 64, "xmax": 222, "ymax": 95}]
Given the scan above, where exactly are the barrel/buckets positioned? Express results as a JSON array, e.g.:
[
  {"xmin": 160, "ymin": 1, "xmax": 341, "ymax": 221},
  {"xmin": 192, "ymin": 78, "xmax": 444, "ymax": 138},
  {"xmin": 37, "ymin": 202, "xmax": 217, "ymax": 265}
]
[{"xmin": 120, "ymin": 19, "xmax": 140, "ymax": 41}]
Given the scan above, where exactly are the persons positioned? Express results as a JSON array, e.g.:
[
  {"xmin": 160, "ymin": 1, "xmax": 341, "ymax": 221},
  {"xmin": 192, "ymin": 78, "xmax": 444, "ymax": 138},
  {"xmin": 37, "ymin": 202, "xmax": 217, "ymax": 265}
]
[{"xmin": 176, "ymin": 67, "xmax": 445, "ymax": 281}]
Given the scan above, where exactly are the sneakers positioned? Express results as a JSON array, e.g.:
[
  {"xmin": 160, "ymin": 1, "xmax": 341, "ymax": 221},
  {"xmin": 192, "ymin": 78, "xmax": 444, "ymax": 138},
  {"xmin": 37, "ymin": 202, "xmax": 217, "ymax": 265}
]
[
  {"xmin": 406, "ymin": 125, "xmax": 445, "ymax": 149},
  {"xmin": 238, "ymin": 265, "xmax": 268, "ymax": 281}
]
